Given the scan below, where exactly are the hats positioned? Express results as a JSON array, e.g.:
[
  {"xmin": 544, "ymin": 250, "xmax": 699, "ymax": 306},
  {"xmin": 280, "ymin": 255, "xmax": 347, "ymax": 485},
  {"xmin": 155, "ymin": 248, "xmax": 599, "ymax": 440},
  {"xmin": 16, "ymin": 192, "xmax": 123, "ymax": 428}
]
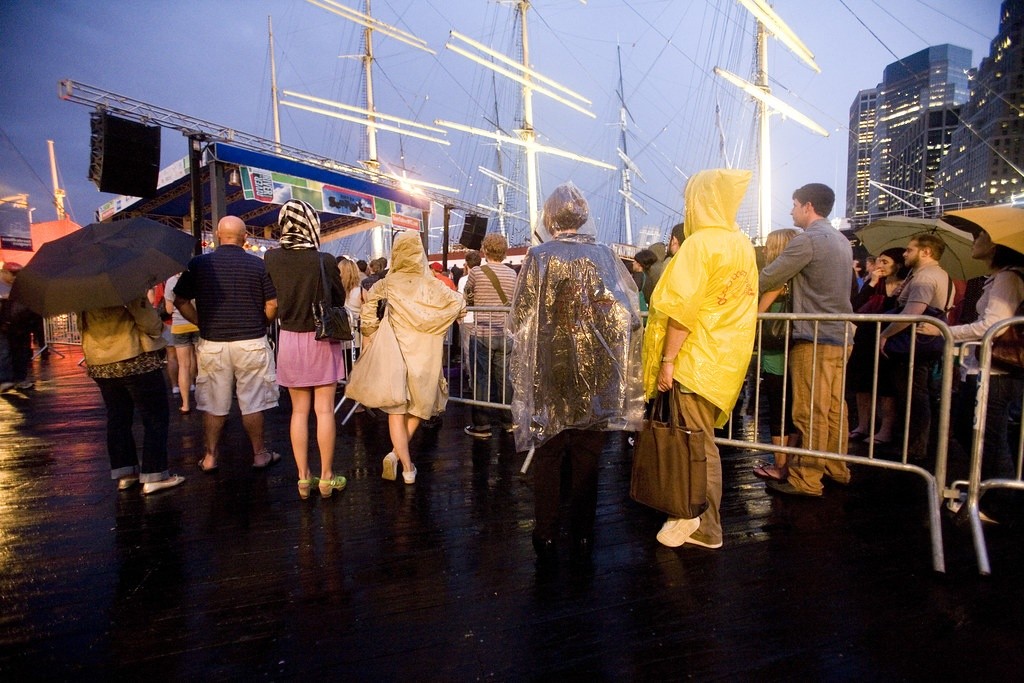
[{"xmin": 3, "ymin": 262, "xmax": 22, "ymax": 272}]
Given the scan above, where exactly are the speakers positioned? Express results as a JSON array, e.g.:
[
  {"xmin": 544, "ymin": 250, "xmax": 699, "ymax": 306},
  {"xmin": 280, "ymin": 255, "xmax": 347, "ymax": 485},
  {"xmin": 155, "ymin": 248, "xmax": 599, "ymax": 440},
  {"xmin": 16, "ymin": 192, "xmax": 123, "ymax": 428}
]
[
  {"xmin": 458, "ymin": 217, "xmax": 489, "ymax": 252},
  {"xmin": 87, "ymin": 114, "xmax": 161, "ymax": 199}
]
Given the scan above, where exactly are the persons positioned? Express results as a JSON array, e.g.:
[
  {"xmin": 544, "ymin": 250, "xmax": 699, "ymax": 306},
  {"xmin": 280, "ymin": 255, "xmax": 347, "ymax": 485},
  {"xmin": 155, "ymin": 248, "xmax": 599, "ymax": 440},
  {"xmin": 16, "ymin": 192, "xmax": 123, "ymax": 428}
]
[
  {"xmin": 641, "ymin": 169, "xmax": 760, "ymax": 548},
  {"xmin": 505, "ymin": 185, "xmax": 641, "ymax": 553},
  {"xmin": 0, "ymin": 184, "xmax": 1024, "ymax": 501}
]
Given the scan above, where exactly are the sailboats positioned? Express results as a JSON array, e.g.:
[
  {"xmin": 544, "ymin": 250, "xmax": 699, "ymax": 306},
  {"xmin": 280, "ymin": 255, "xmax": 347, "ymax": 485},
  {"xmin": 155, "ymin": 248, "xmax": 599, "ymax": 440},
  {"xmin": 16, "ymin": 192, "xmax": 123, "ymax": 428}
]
[{"xmin": 261, "ymin": 0, "xmax": 1024, "ymax": 281}]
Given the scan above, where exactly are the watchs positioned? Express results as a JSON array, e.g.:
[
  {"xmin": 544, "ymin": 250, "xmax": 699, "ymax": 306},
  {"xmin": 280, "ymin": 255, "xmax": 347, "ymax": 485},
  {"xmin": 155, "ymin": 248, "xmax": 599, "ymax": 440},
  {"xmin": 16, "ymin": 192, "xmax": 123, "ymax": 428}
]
[{"xmin": 660, "ymin": 354, "xmax": 674, "ymax": 363}]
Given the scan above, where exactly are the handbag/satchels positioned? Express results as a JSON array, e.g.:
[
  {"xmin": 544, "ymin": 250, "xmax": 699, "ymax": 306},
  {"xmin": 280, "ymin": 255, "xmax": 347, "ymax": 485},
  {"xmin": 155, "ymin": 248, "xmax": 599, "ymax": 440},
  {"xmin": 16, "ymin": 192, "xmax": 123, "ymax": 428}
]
[
  {"xmin": 879, "ymin": 274, "xmax": 945, "ymax": 356},
  {"xmin": 344, "ymin": 278, "xmax": 407, "ymax": 409},
  {"xmin": 755, "ymin": 283, "xmax": 795, "ymax": 352},
  {"xmin": 630, "ymin": 383, "xmax": 709, "ymax": 519},
  {"xmin": 975, "ymin": 269, "xmax": 1024, "ymax": 376},
  {"xmin": 311, "ymin": 252, "xmax": 354, "ymax": 342},
  {"xmin": 357, "ymin": 298, "xmax": 388, "ymax": 333}
]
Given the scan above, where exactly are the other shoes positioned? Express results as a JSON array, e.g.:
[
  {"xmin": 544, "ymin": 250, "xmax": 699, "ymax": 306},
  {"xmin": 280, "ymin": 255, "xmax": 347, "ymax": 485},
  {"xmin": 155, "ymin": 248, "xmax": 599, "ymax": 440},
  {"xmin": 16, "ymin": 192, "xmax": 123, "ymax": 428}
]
[
  {"xmin": 506, "ymin": 428, "xmax": 512, "ymax": 433},
  {"xmin": 403, "ymin": 463, "xmax": 417, "ymax": 484},
  {"xmin": 765, "ymin": 479, "xmax": 802, "ymax": 495},
  {"xmin": 143, "ymin": 474, "xmax": 186, "ymax": 494},
  {"xmin": 118, "ymin": 476, "xmax": 140, "ymax": 490},
  {"xmin": 464, "ymin": 425, "xmax": 492, "ymax": 437},
  {"xmin": 657, "ymin": 516, "xmax": 700, "ymax": 548},
  {"xmin": 173, "ymin": 384, "xmax": 195, "ymax": 415},
  {"xmin": 685, "ymin": 536, "xmax": 723, "ymax": 549},
  {"xmin": 382, "ymin": 452, "xmax": 397, "ymax": 481},
  {"xmin": 14, "ymin": 381, "xmax": 35, "ymax": 390}
]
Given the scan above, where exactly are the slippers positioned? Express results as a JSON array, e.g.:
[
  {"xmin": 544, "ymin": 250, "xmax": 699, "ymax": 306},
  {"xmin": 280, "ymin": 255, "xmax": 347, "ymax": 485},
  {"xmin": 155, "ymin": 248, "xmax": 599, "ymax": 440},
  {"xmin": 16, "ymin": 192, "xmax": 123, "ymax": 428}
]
[
  {"xmin": 198, "ymin": 457, "xmax": 218, "ymax": 472},
  {"xmin": 253, "ymin": 451, "xmax": 281, "ymax": 468},
  {"xmin": 865, "ymin": 437, "xmax": 891, "ymax": 445},
  {"xmin": 755, "ymin": 465, "xmax": 786, "ymax": 481},
  {"xmin": 848, "ymin": 430, "xmax": 866, "ymax": 440}
]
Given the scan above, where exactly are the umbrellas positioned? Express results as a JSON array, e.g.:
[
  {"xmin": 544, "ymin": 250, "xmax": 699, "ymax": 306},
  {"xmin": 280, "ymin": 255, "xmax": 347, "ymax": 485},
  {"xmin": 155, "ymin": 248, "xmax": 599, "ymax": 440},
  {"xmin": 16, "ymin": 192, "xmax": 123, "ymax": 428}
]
[
  {"xmin": 854, "ymin": 216, "xmax": 992, "ymax": 283},
  {"xmin": 10, "ymin": 218, "xmax": 200, "ymax": 316},
  {"xmin": 944, "ymin": 203, "xmax": 1023, "ymax": 261}
]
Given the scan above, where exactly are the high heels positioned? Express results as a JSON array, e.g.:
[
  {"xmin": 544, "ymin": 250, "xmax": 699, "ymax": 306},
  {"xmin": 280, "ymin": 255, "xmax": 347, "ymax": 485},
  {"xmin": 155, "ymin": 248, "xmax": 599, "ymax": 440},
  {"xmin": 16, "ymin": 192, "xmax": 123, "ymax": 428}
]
[
  {"xmin": 318, "ymin": 475, "xmax": 347, "ymax": 498},
  {"xmin": 298, "ymin": 476, "xmax": 320, "ymax": 500}
]
